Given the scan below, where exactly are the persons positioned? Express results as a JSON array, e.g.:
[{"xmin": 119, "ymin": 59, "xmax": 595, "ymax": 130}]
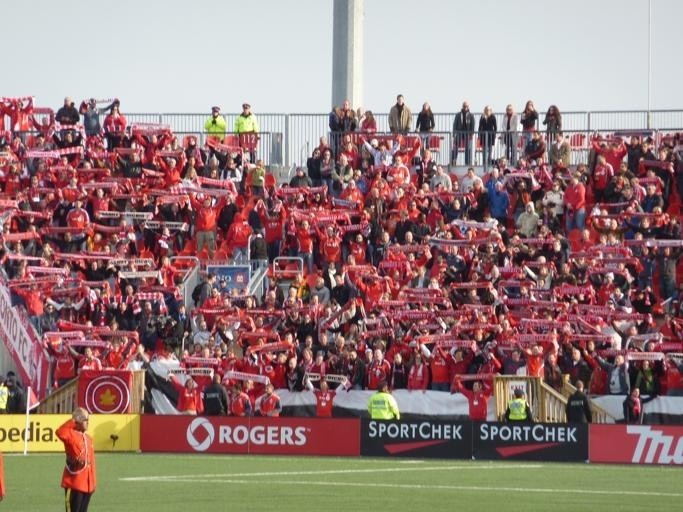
[
  {"xmin": 53, "ymin": 407, "xmax": 96, "ymax": 511},
  {"xmin": 0, "ymin": 95, "xmax": 681, "ymax": 428}
]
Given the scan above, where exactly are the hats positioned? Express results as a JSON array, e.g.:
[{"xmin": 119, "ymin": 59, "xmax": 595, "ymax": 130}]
[{"xmin": 73, "ymin": 407, "xmax": 89, "ymax": 422}]
